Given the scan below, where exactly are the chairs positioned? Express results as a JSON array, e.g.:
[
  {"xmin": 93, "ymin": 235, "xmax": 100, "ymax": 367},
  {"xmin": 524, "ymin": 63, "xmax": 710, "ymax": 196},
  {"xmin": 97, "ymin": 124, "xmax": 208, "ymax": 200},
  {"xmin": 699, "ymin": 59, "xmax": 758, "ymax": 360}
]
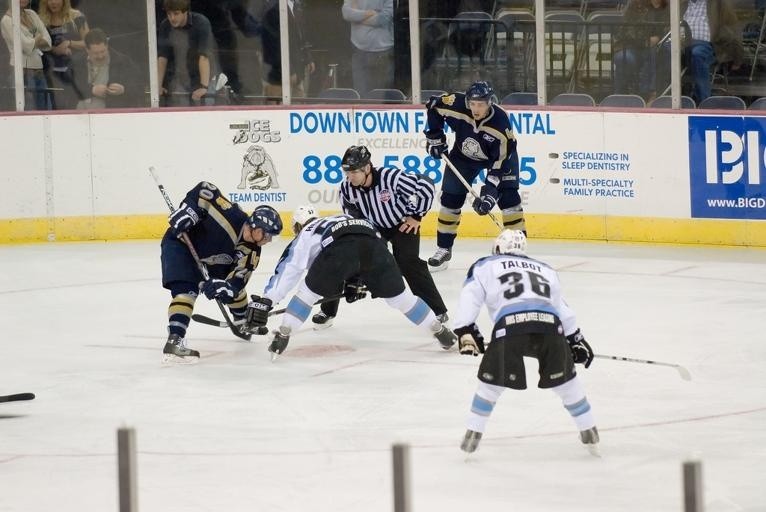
[
  {"xmin": 317, "ymin": 88, "xmax": 360, "ymax": 99},
  {"xmin": 363, "ymin": 89, "xmax": 406, "ymax": 101},
  {"xmin": 599, "ymin": 95, "xmax": 646, "ymax": 108},
  {"xmin": 408, "ymin": 90, "xmax": 447, "ymax": 102},
  {"xmin": 433, "ymin": 0, "xmax": 631, "ymax": 95},
  {"xmin": 501, "ymin": 92, "xmax": 537, "ymax": 105},
  {"xmin": 697, "ymin": 96, "xmax": 747, "ymax": 110},
  {"xmin": 647, "ymin": 95, "xmax": 696, "ymax": 109},
  {"xmin": 689, "ymin": 61, "xmax": 730, "ymax": 100},
  {"xmin": 549, "ymin": 93, "xmax": 595, "ymax": 107},
  {"xmin": 748, "ymin": 98, "xmax": 766, "ymax": 110},
  {"xmin": 741, "ymin": 2, "xmax": 766, "ymax": 81}
]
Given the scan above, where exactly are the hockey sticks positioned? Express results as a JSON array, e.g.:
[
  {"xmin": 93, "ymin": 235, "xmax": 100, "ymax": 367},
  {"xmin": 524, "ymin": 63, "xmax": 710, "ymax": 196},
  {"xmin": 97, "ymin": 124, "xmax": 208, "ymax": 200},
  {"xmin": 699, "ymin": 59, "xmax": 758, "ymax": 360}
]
[
  {"xmin": 192, "ymin": 286, "xmax": 372, "ymax": 327},
  {"xmin": 150, "ymin": 166, "xmax": 253, "ymax": 340},
  {"xmin": 484, "ymin": 342, "xmax": 690, "ymax": 382}
]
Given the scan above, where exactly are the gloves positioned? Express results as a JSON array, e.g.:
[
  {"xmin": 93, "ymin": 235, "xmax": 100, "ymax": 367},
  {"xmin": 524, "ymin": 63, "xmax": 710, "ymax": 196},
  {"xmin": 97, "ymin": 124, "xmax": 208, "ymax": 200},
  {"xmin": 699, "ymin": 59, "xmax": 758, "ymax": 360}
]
[
  {"xmin": 201, "ymin": 278, "xmax": 239, "ymax": 304},
  {"xmin": 345, "ymin": 279, "xmax": 366, "ymax": 303},
  {"xmin": 423, "ymin": 131, "xmax": 449, "ymax": 158},
  {"xmin": 454, "ymin": 324, "xmax": 486, "ymax": 356},
  {"xmin": 566, "ymin": 329, "xmax": 594, "ymax": 369},
  {"xmin": 472, "ymin": 185, "xmax": 500, "ymax": 216},
  {"xmin": 168, "ymin": 198, "xmax": 205, "ymax": 239},
  {"xmin": 247, "ymin": 295, "xmax": 273, "ymax": 326}
]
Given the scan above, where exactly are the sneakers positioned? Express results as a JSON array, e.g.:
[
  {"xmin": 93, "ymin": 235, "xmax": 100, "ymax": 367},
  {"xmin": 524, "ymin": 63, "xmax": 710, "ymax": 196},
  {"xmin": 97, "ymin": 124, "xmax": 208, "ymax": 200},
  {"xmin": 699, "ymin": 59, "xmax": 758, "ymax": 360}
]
[
  {"xmin": 579, "ymin": 426, "xmax": 600, "ymax": 444},
  {"xmin": 313, "ymin": 309, "xmax": 335, "ymax": 324},
  {"xmin": 459, "ymin": 430, "xmax": 482, "ymax": 452},
  {"xmin": 435, "ymin": 326, "xmax": 457, "ymax": 349},
  {"xmin": 268, "ymin": 333, "xmax": 290, "ymax": 355},
  {"xmin": 234, "ymin": 316, "xmax": 269, "ymax": 335},
  {"xmin": 163, "ymin": 332, "xmax": 199, "ymax": 357},
  {"xmin": 437, "ymin": 313, "xmax": 449, "ymax": 322},
  {"xmin": 429, "ymin": 248, "xmax": 452, "ymax": 266}
]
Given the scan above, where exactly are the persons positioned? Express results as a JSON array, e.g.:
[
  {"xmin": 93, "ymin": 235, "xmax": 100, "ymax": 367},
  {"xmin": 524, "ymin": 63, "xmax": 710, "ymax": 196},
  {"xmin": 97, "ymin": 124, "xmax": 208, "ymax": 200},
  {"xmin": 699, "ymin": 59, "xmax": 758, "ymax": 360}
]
[
  {"xmin": 656, "ymin": 0, "xmax": 745, "ymax": 101},
  {"xmin": 423, "ymin": 81, "xmax": 528, "ymax": 268},
  {"xmin": 0, "ymin": 0, "xmax": 142, "ymax": 110},
  {"xmin": 337, "ymin": 144, "xmax": 452, "ymax": 330},
  {"xmin": 156, "ymin": 0, "xmax": 222, "ymax": 104},
  {"xmin": 244, "ymin": 204, "xmax": 457, "ymax": 363},
  {"xmin": 611, "ymin": 0, "xmax": 667, "ymax": 105},
  {"xmin": 234, "ymin": 0, "xmax": 402, "ymax": 107},
  {"xmin": 160, "ymin": 181, "xmax": 283, "ymax": 358},
  {"xmin": 156, "ymin": 0, "xmax": 242, "ymax": 94},
  {"xmin": 453, "ymin": 228, "xmax": 604, "ymax": 466}
]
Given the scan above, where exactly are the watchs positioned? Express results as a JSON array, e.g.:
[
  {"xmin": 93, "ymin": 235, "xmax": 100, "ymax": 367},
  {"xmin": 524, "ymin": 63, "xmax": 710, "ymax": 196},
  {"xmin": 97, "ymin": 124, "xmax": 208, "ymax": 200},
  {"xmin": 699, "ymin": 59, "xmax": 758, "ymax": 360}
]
[{"xmin": 199, "ymin": 83, "xmax": 207, "ymax": 89}]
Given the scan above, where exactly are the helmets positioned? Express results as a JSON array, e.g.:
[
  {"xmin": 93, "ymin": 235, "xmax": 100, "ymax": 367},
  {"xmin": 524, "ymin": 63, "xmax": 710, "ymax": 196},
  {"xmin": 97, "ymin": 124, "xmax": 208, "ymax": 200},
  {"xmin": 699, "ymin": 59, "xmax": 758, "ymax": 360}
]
[
  {"xmin": 291, "ymin": 207, "xmax": 318, "ymax": 228},
  {"xmin": 494, "ymin": 229, "xmax": 526, "ymax": 256},
  {"xmin": 341, "ymin": 146, "xmax": 371, "ymax": 171},
  {"xmin": 465, "ymin": 81, "xmax": 495, "ymax": 101},
  {"xmin": 248, "ymin": 205, "xmax": 283, "ymax": 235}
]
